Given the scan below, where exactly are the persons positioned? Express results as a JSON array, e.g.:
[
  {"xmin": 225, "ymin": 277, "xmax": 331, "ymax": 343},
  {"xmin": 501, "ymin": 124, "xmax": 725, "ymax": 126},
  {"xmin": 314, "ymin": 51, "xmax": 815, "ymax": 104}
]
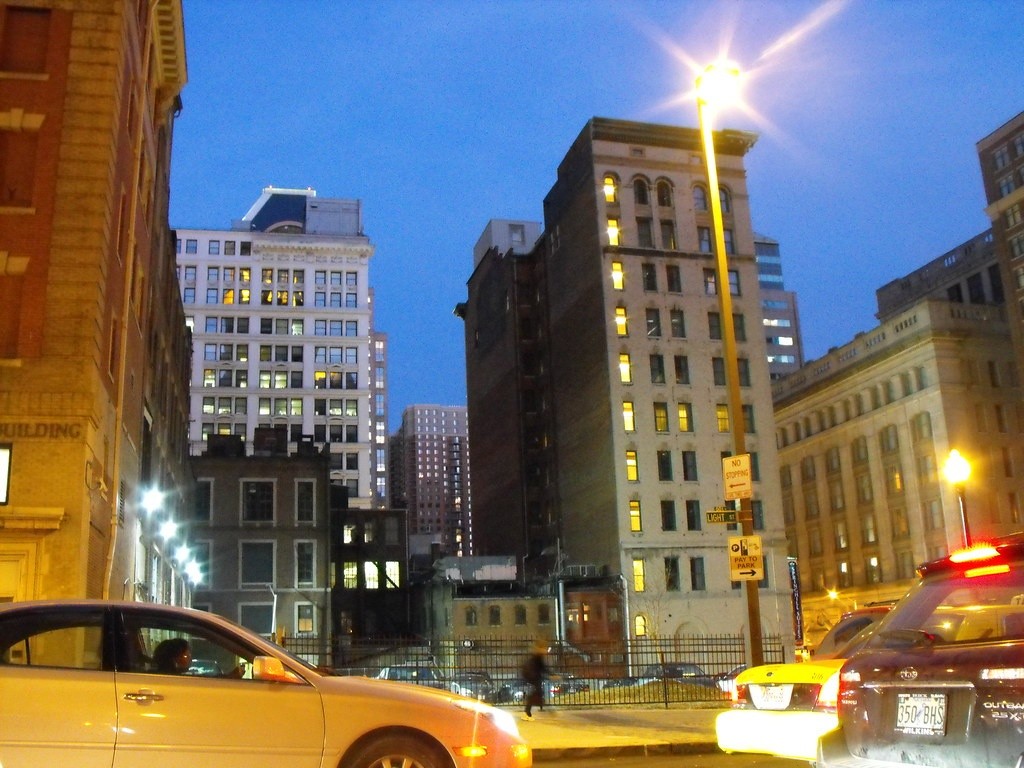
[
  {"xmin": 148, "ymin": 638, "xmax": 245, "ymax": 679},
  {"xmin": 522, "ymin": 654, "xmax": 549, "ymax": 720}
]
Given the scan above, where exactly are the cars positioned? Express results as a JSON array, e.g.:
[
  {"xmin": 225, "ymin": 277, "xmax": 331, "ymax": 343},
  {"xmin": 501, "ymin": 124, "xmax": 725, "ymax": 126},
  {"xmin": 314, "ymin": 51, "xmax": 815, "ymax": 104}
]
[
  {"xmin": 714, "ymin": 594, "xmax": 1024, "ymax": 763},
  {"xmin": 0, "ymin": 598, "xmax": 533, "ymax": 768},
  {"xmin": 371, "ymin": 664, "xmax": 750, "ymax": 703},
  {"xmin": 835, "ymin": 530, "xmax": 1024, "ymax": 767}
]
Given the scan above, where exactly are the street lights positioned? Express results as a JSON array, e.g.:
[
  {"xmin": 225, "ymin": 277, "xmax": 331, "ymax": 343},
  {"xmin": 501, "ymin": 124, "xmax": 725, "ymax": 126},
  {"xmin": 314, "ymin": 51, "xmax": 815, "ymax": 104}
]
[
  {"xmin": 942, "ymin": 448, "xmax": 970, "ymax": 548},
  {"xmin": 692, "ymin": 61, "xmax": 763, "ymax": 668}
]
